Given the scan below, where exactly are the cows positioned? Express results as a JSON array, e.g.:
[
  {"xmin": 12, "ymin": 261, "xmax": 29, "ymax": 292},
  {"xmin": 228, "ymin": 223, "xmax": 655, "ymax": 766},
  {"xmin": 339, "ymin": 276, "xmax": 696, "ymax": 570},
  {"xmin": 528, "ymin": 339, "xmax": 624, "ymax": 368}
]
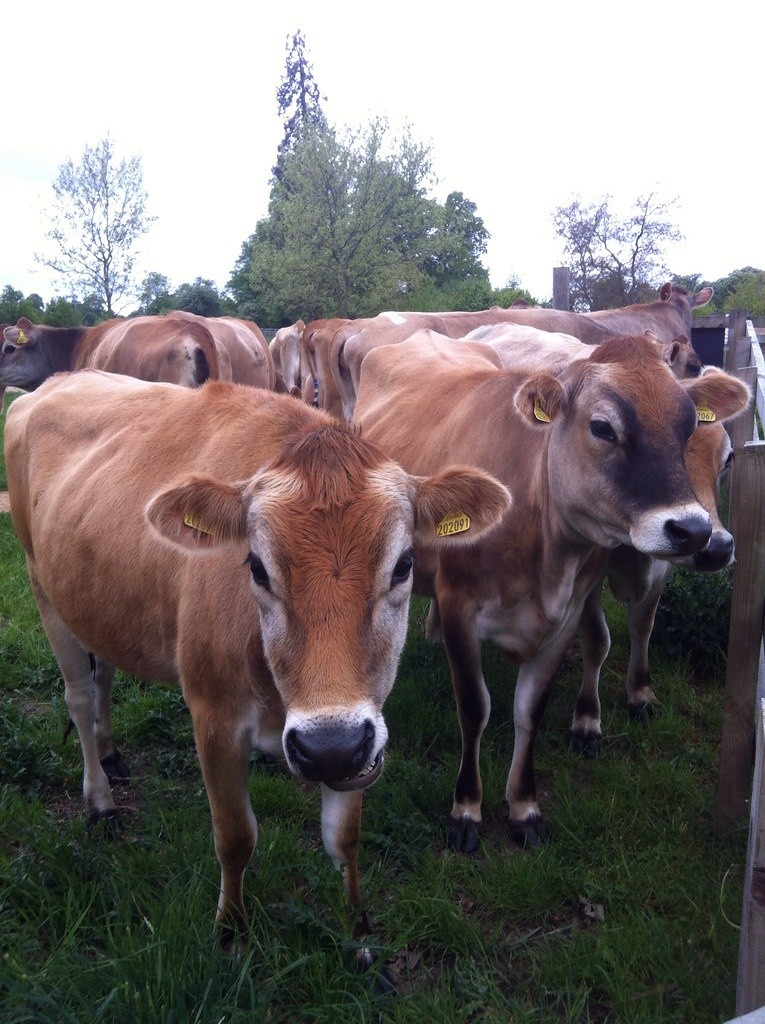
[{"xmin": 0, "ymin": 282, "xmax": 752, "ymax": 978}]
